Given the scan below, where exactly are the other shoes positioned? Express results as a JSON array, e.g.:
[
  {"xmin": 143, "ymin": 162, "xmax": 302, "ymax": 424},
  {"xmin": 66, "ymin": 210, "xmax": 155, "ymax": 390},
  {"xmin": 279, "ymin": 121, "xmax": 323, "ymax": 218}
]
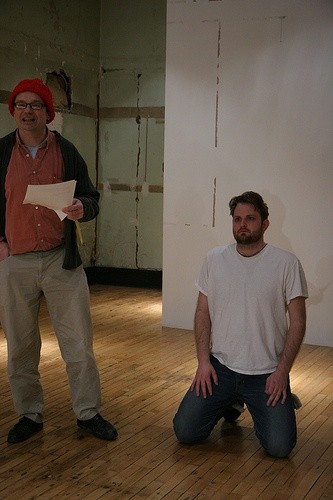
[
  {"xmin": 223, "ymin": 400, "xmax": 244, "ymax": 422},
  {"xmin": 290, "ymin": 393, "xmax": 302, "ymax": 410}
]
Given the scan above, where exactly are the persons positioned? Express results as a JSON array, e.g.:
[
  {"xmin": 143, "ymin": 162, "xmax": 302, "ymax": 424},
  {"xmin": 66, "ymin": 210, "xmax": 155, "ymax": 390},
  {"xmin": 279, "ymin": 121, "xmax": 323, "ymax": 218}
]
[
  {"xmin": 172, "ymin": 191, "xmax": 310, "ymax": 457},
  {"xmin": 0, "ymin": 78, "xmax": 119, "ymax": 445}
]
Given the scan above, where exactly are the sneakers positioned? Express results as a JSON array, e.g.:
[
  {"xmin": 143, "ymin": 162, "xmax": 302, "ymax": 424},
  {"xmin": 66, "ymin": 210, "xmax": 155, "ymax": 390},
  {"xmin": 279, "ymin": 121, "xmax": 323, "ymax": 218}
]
[
  {"xmin": 7, "ymin": 416, "xmax": 43, "ymax": 443},
  {"xmin": 76, "ymin": 413, "xmax": 118, "ymax": 441}
]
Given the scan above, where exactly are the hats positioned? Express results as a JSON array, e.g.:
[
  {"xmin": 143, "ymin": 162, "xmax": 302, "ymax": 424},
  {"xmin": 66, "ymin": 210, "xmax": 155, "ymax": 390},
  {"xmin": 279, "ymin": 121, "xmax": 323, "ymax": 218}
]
[{"xmin": 8, "ymin": 78, "xmax": 55, "ymax": 124}]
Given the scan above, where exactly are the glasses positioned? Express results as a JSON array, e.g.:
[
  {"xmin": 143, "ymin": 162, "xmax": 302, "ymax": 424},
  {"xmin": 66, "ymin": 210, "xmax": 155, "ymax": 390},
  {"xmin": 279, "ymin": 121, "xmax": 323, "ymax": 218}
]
[{"xmin": 13, "ymin": 102, "xmax": 46, "ymax": 110}]
